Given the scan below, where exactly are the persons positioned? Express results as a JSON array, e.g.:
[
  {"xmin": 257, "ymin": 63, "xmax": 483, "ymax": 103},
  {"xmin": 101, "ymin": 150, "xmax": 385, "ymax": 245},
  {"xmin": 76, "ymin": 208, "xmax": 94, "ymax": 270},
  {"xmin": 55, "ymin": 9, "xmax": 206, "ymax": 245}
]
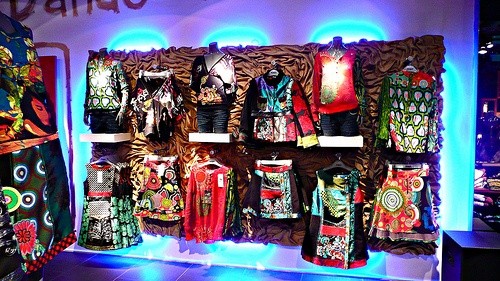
[
  {"xmin": 82, "ymin": 46, "xmax": 129, "ymax": 132},
  {"xmin": 188, "ymin": 42, "xmax": 236, "ymax": 134},
  {"xmin": 311, "ymin": 34, "xmax": 362, "ymax": 137}
]
